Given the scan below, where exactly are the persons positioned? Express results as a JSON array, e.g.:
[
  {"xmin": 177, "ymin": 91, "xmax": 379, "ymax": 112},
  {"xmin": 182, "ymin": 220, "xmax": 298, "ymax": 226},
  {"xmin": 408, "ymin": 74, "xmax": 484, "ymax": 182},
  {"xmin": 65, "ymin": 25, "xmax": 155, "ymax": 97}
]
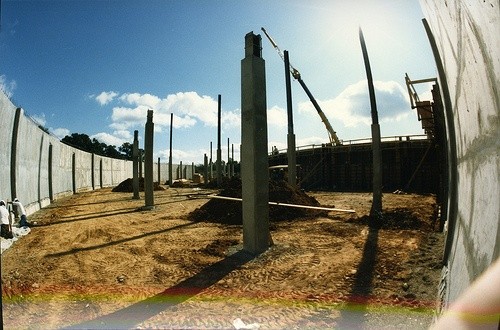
[
  {"xmin": 7, "ymin": 198, "xmax": 30, "ymax": 227},
  {"xmin": 0, "ymin": 200, "xmax": 15, "ymax": 240}
]
